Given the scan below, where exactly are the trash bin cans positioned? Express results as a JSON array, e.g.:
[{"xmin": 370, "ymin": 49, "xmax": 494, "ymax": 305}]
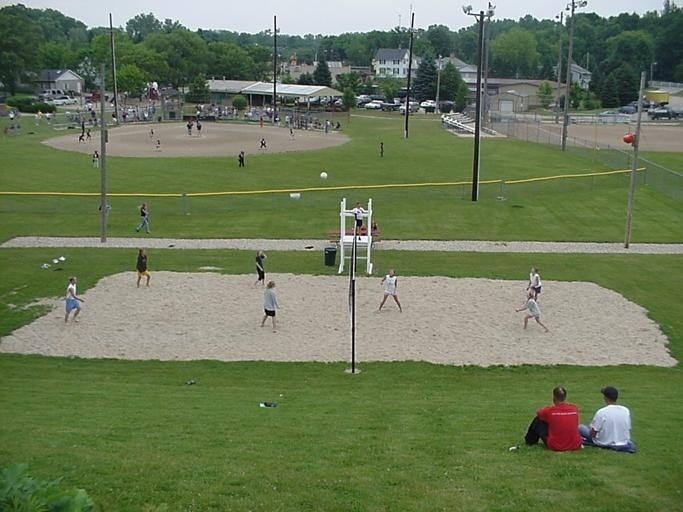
[{"xmin": 324, "ymin": 247, "xmax": 336, "ymax": 266}]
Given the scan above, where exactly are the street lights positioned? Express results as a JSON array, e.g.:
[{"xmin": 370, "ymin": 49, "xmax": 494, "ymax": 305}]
[
  {"xmin": 554, "ymin": 9, "xmax": 563, "ymax": 124},
  {"xmin": 559, "ymin": 0, "xmax": 587, "ymax": 149},
  {"xmin": 650, "ymin": 61, "xmax": 657, "ymax": 80},
  {"xmin": 454, "ymin": 1, "xmax": 497, "ymax": 201},
  {"xmin": 434, "ymin": 54, "xmax": 443, "ymax": 113}
]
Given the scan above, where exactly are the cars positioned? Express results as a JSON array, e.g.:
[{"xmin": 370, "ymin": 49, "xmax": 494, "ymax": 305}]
[
  {"xmin": 619, "ymin": 99, "xmax": 680, "ymax": 120},
  {"xmin": 39, "ymin": 88, "xmax": 78, "ymax": 106},
  {"xmin": 677, "ymin": 109, "xmax": 682, "ymax": 118},
  {"xmin": 289, "ymin": 88, "xmax": 434, "ymax": 114},
  {"xmin": 598, "ymin": 109, "xmax": 630, "ymax": 119}
]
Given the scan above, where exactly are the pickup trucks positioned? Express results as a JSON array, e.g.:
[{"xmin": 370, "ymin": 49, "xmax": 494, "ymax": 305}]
[{"xmin": 84, "ymin": 92, "xmax": 108, "ymax": 104}]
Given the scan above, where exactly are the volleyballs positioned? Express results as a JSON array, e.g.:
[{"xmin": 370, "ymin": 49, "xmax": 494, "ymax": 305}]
[{"xmin": 319, "ymin": 171, "xmax": 328, "ymax": 179}]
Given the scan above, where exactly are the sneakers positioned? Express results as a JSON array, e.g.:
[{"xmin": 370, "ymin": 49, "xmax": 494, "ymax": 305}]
[{"xmin": 507, "ymin": 444, "xmax": 526, "ymax": 451}]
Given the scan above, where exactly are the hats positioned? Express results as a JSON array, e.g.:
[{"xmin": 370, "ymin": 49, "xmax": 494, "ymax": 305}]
[{"xmin": 600, "ymin": 386, "xmax": 619, "ymax": 400}]
